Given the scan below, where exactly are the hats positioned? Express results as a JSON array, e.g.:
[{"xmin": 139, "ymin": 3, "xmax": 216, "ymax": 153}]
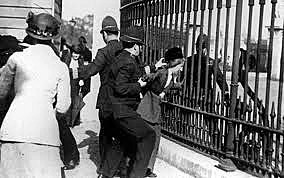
[
  {"xmin": 72, "ymin": 44, "xmax": 82, "ymax": 53},
  {"xmin": 165, "ymin": 47, "xmax": 183, "ymax": 60},
  {"xmin": 25, "ymin": 11, "xmax": 60, "ymax": 40},
  {"xmin": 119, "ymin": 28, "xmax": 145, "ymax": 43},
  {"xmin": 100, "ymin": 16, "xmax": 119, "ymax": 32}
]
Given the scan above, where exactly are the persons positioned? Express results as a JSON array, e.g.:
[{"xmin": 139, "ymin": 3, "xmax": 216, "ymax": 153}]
[
  {"xmin": 79, "ymin": 15, "xmax": 126, "ymax": 173},
  {"xmin": 0, "ymin": 12, "xmax": 80, "ymax": 178},
  {"xmin": 78, "ymin": 35, "xmax": 92, "ymax": 111},
  {"xmin": 61, "ymin": 49, "xmax": 84, "ymax": 127},
  {"xmin": 180, "ymin": 33, "xmax": 231, "ymax": 151},
  {"xmin": 134, "ymin": 47, "xmax": 185, "ymax": 177},
  {"xmin": 97, "ymin": 25, "xmax": 167, "ymax": 177}
]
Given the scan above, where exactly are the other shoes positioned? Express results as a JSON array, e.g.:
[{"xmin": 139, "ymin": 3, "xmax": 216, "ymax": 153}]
[
  {"xmin": 63, "ymin": 156, "xmax": 80, "ymax": 170},
  {"xmin": 97, "ymin": 166, "xmax": 157, "ymax": 178}
]
